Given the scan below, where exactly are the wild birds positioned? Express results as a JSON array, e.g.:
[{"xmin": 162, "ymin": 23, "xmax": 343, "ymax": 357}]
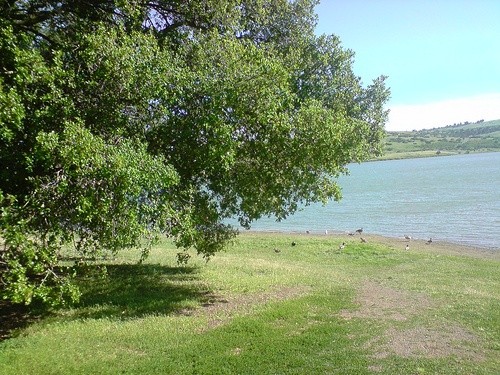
[{"xmin": 273, "ymin": 227, "xmax": 433, "ymax": 256}]
